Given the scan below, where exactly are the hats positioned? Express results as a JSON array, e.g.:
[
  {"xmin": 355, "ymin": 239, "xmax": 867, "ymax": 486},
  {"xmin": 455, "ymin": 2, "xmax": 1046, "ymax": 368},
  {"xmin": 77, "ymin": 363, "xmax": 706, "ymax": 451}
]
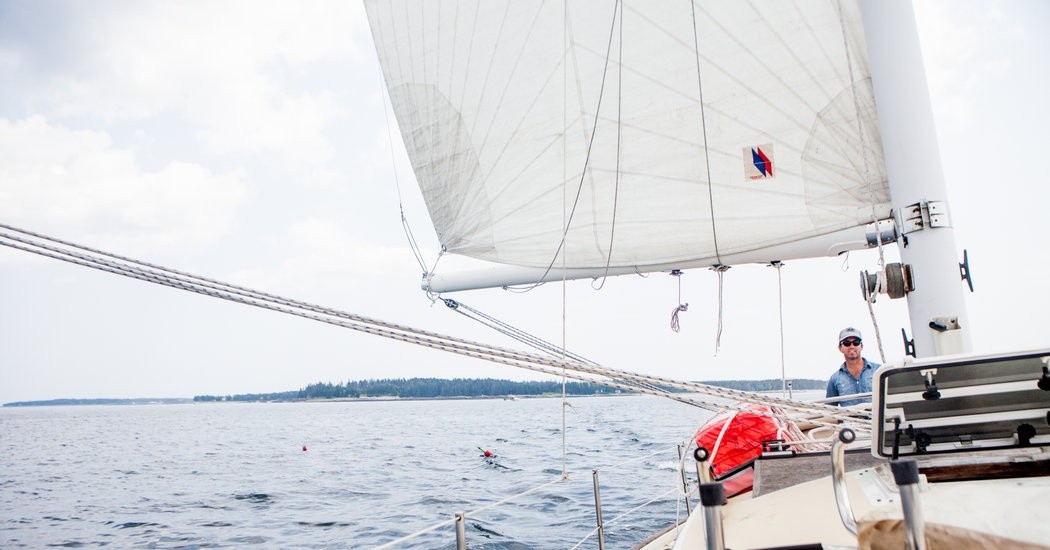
[{"xmin": 839, "ymin": 327, "xmax": 862, "ymax": 341}]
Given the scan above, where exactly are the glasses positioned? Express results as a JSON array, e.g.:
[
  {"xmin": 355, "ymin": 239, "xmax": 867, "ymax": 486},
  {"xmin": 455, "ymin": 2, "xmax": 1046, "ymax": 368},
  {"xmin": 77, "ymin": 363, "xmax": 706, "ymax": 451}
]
[{"xmin": 840, "ymin": 340, "xmax": 861, "ymax": 346}]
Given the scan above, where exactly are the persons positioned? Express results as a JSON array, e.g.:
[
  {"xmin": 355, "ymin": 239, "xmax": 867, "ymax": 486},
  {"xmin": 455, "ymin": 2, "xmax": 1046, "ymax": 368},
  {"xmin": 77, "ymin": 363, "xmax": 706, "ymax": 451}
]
[{"xmin": 824, "ymin": 327, "xmax": 882, "ymax": 410}]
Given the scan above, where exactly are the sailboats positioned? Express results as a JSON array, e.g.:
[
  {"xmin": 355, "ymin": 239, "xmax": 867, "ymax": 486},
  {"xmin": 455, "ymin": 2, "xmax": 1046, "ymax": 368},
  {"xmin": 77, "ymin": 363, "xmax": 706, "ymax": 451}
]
[{"xmin": 357, "ymin": 0, "xmax": 1050, "ymax": 549}]
